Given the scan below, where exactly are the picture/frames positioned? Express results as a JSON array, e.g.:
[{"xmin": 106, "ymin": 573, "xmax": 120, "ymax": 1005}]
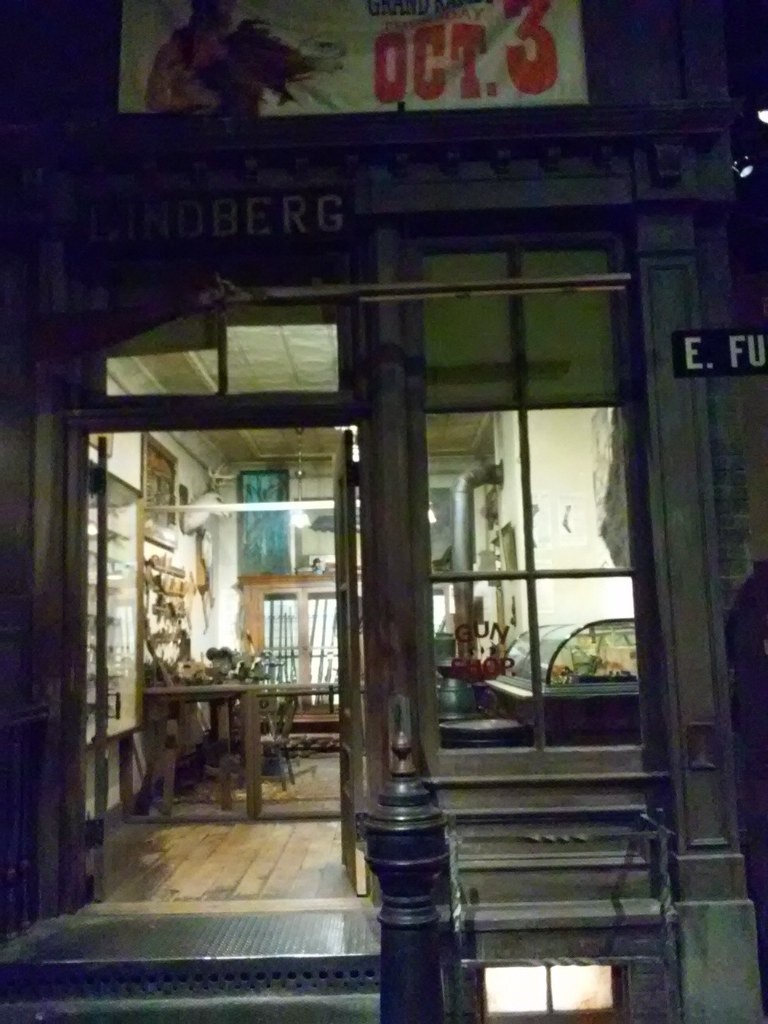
[
  {"xmin": 140, "ymin": 430, "xmax": 177, "ymax": 551},
  {"xmin": 237, "ymin": 469, "xmax": 290, "ymax": 572}
]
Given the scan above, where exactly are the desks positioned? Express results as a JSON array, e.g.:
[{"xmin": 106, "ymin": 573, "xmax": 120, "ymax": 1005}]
[{"xmin": 143, "ymin": 683, "xmax": 338, "ymax": 822}]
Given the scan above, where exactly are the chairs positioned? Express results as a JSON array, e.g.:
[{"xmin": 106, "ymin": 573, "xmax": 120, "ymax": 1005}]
[{"xmin": 239, "ymin": 696, "xmax": 300, "ymax": 791}]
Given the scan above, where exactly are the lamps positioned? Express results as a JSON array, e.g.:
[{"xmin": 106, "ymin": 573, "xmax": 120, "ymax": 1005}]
[{"xmin": 291, "ymin": 427, "xmax": 311, "ymax": 529}]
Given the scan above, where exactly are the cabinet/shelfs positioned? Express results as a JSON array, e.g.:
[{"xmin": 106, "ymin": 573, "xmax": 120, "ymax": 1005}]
[
  {"xmin": 237, "ymin": 574, "xmax": 452, "ymax": 725},
  {"xmin": 86, "ymin": 458, "xmax": 143, "ymax": 750}
]
